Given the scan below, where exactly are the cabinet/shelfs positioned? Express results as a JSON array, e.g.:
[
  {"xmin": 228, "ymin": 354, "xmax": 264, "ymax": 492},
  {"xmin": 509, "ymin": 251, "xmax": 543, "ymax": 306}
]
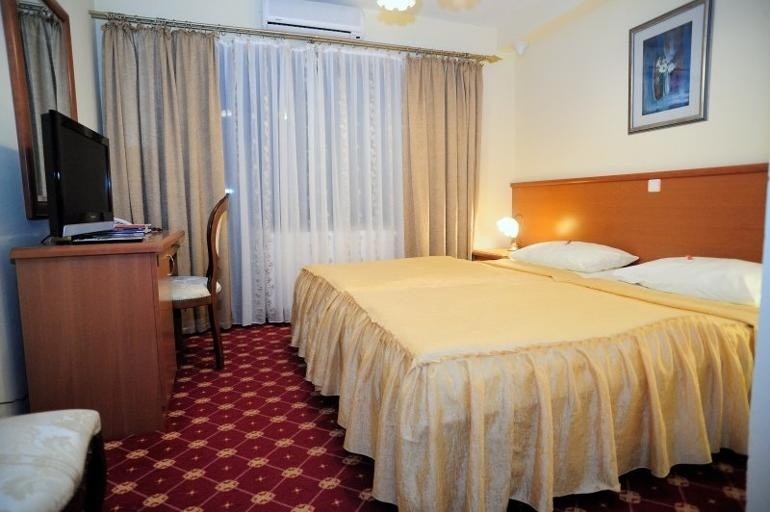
[{"xmin": 11, "ymin": 229, "xmax": 186, "ymax": 437}]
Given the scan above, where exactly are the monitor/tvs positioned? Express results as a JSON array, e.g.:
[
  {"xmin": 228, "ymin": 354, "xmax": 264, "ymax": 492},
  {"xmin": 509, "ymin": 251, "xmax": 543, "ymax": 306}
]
[{"xmin": 40, "ymin": 108, "xmax": 115, "ymax": 237}]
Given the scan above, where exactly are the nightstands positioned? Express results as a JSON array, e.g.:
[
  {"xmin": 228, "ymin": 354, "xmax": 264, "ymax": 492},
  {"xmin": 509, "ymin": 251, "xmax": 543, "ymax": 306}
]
[{"xmin": 472, "ymin": 245, "xmax": 511, "ymax": 265}]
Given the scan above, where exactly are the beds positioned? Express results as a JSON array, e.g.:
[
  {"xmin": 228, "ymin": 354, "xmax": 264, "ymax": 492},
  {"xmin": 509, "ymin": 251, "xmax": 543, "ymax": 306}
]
[{"xmin": 289, "ymin": 163, "xmax": 769, "ymax": 511}]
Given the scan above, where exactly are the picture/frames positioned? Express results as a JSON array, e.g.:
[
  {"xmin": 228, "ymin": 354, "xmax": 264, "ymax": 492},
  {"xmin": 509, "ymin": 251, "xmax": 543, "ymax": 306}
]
[{"xmin": 627, "ymin": 0, "xmax": 710, "ymax": 134}]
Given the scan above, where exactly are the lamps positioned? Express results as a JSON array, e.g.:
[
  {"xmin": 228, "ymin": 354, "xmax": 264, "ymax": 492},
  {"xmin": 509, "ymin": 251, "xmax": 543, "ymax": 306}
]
[{"xmin": 496, "ymin": 214, "xmax": 524, "ymax": 251}]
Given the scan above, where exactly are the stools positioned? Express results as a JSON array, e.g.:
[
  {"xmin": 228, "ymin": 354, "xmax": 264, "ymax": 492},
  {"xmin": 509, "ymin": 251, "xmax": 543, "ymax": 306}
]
[{"xmin": 0, "ymin": 409, "xmax": 111, "ymax": 511}]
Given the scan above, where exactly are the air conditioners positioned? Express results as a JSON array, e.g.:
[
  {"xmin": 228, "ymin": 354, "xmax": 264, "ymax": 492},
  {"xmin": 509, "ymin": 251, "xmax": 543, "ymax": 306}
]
[{"xmin": 261, "ymin": 0, "xmax": 366, "ymax": 40}]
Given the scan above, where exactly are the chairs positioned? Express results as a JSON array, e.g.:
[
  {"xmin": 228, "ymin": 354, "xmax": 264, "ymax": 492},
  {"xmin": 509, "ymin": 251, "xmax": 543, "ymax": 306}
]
[{"xmin": 166, "ymin": 192, "xmax": 231, "ymax": 372}]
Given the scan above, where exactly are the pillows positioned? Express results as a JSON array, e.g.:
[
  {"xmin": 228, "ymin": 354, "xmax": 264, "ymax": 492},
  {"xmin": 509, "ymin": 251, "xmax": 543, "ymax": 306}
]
[
  {"xmin": 614, "ymin": 254, "xmax": 760, "ymax": 307},
  {"xmin": 510, "ymin": 241, "xmax": 640, "ymax": 274}
]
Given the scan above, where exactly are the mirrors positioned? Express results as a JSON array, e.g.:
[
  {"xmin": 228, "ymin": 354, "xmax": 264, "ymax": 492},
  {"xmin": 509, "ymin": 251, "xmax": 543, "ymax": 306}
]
[{"xmin": 0, "ymin": 1, "xmax": 78, "ymax": 220}]
